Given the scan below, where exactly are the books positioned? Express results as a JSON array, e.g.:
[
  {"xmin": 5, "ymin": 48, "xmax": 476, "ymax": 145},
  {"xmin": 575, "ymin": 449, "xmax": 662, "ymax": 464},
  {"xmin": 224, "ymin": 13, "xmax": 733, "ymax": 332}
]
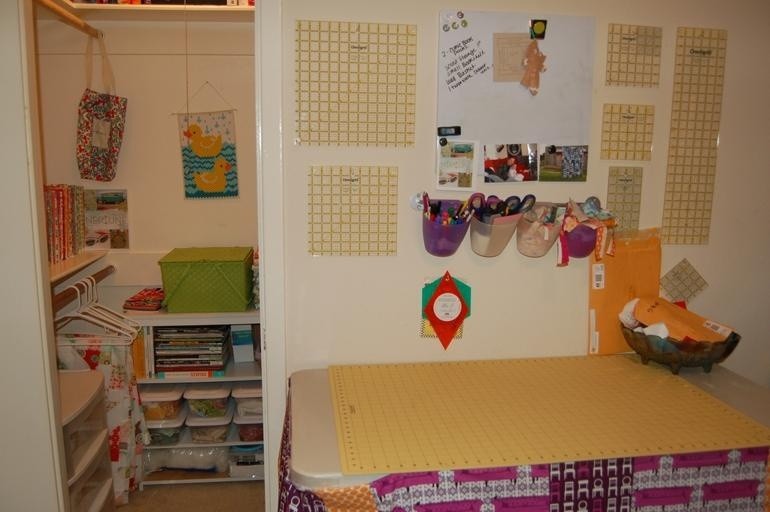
[
  {"xmin": 44, "ymin": 183, "xmax": 86, "ymax": 266},
  {"xmin": 131, "ymin": 325, "xmax": 233, "ymax": 379}
]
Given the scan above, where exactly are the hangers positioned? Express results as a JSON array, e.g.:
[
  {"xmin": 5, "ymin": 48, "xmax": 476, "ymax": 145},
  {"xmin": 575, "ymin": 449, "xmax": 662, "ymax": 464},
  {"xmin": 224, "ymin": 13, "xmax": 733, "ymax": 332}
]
[{"xmin": 52, "ymin": 276, "xmax": 141, "ymax": 346}]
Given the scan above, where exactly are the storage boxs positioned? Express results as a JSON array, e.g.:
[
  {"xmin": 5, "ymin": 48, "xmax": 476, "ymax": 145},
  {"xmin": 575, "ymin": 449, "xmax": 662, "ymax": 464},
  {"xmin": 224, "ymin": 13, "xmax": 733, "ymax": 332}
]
[
  {"xmin": 136, "ymin": 380, "xmax": 263, "ymax": 446},
  {"xmin": 158, "ymin": 247, "xmax": 255, "ymax": 313}
]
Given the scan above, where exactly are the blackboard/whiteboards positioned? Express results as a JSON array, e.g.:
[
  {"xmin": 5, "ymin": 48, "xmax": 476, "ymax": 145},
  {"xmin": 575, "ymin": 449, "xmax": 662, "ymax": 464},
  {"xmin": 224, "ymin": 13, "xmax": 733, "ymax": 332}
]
[{"xmin": 438, "ymin": 7, "xmax": 599, "ymax": 148}]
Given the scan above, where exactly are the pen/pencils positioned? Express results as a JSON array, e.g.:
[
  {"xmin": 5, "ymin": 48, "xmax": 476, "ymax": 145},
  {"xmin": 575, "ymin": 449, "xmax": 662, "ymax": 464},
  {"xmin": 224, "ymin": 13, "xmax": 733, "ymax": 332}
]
[{"xmin": 422, "ymin": 192, "xmax": 475, "ymax": 224}]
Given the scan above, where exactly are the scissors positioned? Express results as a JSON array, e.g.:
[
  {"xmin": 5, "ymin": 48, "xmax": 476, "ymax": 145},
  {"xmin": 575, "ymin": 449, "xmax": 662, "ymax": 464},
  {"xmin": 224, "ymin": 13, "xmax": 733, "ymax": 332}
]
[{"xmin": 469, "ymin": 193, "xmax": 536, "ymax": 221}]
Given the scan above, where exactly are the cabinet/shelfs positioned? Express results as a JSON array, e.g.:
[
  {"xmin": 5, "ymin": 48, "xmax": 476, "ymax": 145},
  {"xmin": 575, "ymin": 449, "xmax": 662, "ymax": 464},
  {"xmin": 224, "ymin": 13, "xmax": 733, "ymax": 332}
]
[
  {"xmin": 121, "ymin": 308, "xmax": 264, "ymax": 491},
  {"xmin": 59, "ymin": 369, "xmax": 117, "ymax": 512}
]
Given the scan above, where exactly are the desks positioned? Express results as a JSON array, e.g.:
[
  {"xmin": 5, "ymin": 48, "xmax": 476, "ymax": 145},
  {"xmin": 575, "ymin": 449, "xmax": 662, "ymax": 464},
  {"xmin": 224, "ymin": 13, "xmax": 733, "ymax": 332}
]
[{"xmin": 285, "ymin": 351, "xmax": 770, "ymax": 512}]
